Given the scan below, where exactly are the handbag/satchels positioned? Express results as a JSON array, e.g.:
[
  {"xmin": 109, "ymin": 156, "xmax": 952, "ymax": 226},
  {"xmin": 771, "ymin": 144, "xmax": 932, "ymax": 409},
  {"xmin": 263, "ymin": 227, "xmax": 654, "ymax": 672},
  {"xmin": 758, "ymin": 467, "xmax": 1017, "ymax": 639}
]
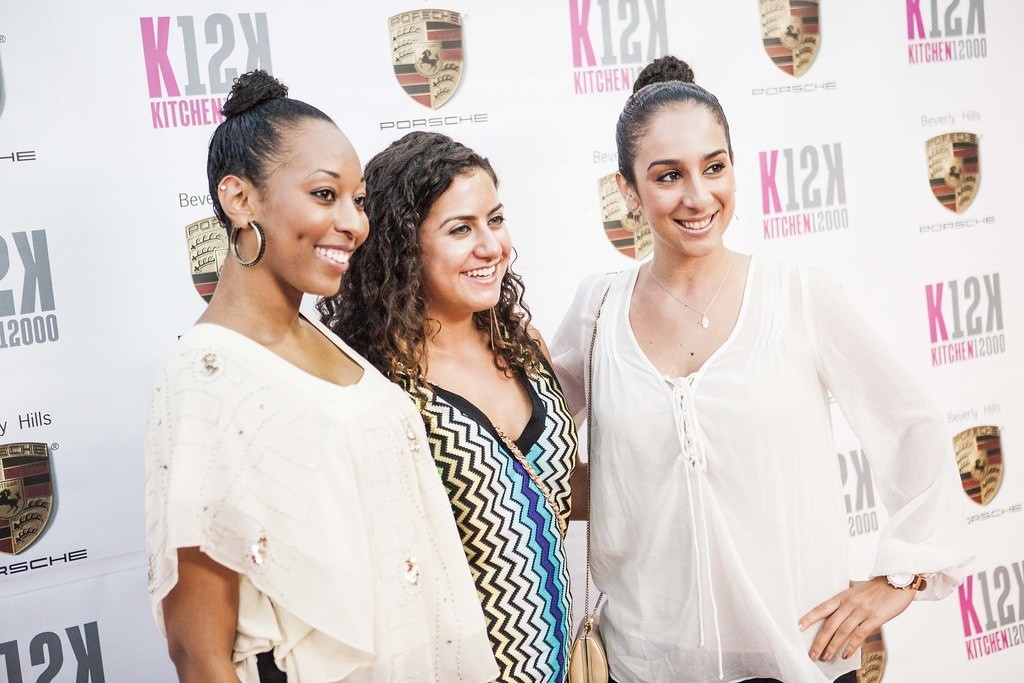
[{"xmin": 568, "ymin": 612, "xmax": 609, "ymax": 683}]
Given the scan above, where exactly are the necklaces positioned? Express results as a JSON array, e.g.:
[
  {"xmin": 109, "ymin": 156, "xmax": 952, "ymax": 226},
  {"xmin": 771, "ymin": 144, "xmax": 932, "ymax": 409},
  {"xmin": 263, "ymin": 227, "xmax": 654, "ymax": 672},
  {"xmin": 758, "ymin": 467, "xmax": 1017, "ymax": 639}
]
[{"xmin": 650, "ymin": 257, "xmax": 732, "ymax": 329}]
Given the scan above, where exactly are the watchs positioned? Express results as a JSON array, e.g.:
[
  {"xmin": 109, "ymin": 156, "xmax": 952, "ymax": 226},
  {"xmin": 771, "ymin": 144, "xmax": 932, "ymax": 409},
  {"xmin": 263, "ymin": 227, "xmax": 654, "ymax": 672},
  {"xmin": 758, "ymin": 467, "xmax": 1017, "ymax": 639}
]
[{"xmin": 886, "ymin": 572, "xmax": 929, "ymax": 590}]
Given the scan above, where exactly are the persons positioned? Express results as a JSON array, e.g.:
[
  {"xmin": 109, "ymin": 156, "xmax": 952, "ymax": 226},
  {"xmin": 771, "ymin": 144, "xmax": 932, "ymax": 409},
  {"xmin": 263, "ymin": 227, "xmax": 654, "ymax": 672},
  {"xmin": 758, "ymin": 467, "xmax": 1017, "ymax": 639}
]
[
  {"xmin": 316, "ymin": 131, "xmax": 580, "ymax": 683},
  {"xmin": 549, "ymin": 54, "xmax": 975, "ymax": 683},
  {"xmin": 144, "ymin": 69, "xmax": 502, "ymax": 683}
]
